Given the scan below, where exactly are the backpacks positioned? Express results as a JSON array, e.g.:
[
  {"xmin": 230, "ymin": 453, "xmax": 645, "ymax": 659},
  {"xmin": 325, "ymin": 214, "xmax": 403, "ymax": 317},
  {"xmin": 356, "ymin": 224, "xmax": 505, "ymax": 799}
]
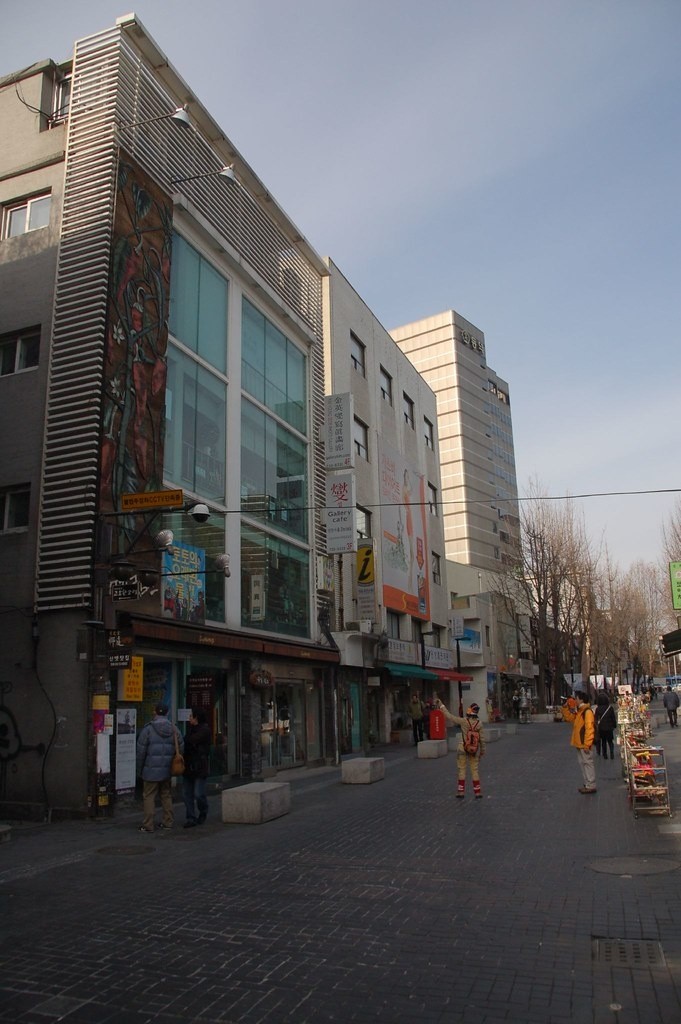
[{"xmin": 462, "ymin": 718, "xmax": 480, "ymax": 754}]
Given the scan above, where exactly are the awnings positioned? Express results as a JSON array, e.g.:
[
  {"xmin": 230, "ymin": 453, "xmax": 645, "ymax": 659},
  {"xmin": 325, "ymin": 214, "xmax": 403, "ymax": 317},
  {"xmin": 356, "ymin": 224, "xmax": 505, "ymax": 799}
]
[{"xmin": 386, "ymin": 663, "xmax": 474, "ymax": 681}]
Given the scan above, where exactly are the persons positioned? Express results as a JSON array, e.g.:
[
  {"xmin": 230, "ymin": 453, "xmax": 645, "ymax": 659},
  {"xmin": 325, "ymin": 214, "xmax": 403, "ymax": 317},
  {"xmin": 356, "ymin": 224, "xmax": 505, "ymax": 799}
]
[
  {"xmin": 181, "ymin": 707, "xmax": 210, "ymax": 826},
  {"xmin": 436, "ymin": 700, "xmax": 486, "ymax": 797},
  {"xmin": 407, "ymin": 684, "xmax": 679, "ymax": 759},
  {"xmin": 136, "ymin": 702, "xmax": 185, "ymax": 831},
  {"xmin": 562, "ymin": 692, "xmax": 598, "ymax": 793},
  {"xmin": 403, "ymin": 469, "xmax": 413, "ymax": 592}
]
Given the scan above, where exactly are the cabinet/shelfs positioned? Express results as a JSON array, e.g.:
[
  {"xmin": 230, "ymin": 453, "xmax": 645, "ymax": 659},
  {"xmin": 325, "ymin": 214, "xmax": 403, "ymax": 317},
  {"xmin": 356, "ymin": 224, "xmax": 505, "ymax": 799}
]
[{"xmin": 614, "ymin": 684, "xmax": 674, "ymax": 819}]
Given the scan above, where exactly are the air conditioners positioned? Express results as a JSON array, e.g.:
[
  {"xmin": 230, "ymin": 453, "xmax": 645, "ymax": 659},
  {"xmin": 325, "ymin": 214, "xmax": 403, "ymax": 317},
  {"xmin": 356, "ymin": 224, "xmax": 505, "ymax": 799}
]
[{"xmin": 345, "ymin": 620, "xmax": 372, "ymax": 634}]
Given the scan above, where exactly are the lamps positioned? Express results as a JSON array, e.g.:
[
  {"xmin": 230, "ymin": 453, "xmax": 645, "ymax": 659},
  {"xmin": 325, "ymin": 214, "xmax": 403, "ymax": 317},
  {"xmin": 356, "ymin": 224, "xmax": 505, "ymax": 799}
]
[
  {"xmin": 112, "ymin": 530, "xmax": 174, "ymax": 558},
  {"xmin": 159, "ymin": 553, "xmax": 231, "ymax": 578},
  {"xmin": 119, "ymin": 103, "xmax": 191, "ymax": 132},
  {"xmin": 171, "ymin": 162, "xmax": 237, "ymax": 185}
]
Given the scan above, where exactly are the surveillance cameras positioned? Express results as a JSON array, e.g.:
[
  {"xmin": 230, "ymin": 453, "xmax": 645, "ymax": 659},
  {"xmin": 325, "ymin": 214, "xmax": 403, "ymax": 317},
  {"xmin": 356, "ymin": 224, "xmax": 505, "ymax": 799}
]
[{"xmin": 191, "ymin": 504, "xmax": 211, "ymax": 523}]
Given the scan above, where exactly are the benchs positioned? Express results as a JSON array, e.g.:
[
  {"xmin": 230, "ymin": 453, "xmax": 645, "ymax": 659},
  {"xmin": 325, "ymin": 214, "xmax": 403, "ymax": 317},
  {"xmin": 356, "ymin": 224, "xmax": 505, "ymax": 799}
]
[
  {"xmin": 222, "ymin": 781, "xmax": 291, "ymax": 824},
  {"xmin": 342, "ymin": 758, "xmax": 385, "ymax": 785},
  {"xmin": 482, "ymin": 729, "xmax": 498, "ymax": 742},
  {"xmin": 507, "ymin": 724, "xmax": 521, "ymax": 734},
  {"xmin": 418, "ymin": 740, "xmax": 448, "ymax": 759},
  {"xmin": 456, "ymin": 733, "xmax": 464, "ymax": 748}
]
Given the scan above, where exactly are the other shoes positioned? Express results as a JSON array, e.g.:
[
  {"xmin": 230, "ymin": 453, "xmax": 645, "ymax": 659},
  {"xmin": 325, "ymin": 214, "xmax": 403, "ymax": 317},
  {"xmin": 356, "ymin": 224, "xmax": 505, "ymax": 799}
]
[
  {"xmin": 578, "ymin": 788, "xmax": 586, "ymax": 792},
  {"xmin": 138, "ymin": 827, "xmax": 153, "ymax": 833},
  {"xmin": 671, "ymin": 723, "xmax": 674, "ymax": 728},
  {"xmin": 674, "ymin": 721, "xmax": 678, "ymax": 727},
  {"xmin": 580, "ymin": 788, "xmax": 596, "ymax": 794},
  {"xmin": 155, "ymin": 823, "xmax": 173, "ymax": 830},
  {"xmin": 420, "ymin": 737, "xmax": 423, "ymax": 741},
  {"xmin": 414, "ymin": 742, "xmax": 416, "ymax": 747}
]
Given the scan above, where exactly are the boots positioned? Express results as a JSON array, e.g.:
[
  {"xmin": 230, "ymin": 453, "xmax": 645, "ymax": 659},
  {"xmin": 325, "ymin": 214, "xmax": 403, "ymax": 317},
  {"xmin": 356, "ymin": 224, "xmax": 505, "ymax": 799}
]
[
  {"xmin": 182, "ymin": 801, "xmax": 197, "ymax": 828},
  {"xmin": 602, "ymin": 740, "xmax": 608, "ymax": 759},
  {"xmin": 609, "ymin": 743, "xmax": 614, "ymax": 760},
  {"xmin": 473, "ymin": 781, "xmax": 483, "ymax": 798},
  {"xmin": 194, "ymin": 795, "xmax": 208, "ymax": 825},
  {"xmin": 455, "ymin": 779, "xmax": 465, "ymax": 800}
]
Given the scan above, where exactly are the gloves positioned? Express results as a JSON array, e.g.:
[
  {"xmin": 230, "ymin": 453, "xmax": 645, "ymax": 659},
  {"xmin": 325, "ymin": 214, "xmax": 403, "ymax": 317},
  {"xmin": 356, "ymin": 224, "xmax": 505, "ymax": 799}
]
[
  {"xmin": 137, "ymin": 768, "xmax": 142, "ymax": 778},
  {"xmin": 434, "ymin": 699, "xmax": 443, "ymax": 706}
]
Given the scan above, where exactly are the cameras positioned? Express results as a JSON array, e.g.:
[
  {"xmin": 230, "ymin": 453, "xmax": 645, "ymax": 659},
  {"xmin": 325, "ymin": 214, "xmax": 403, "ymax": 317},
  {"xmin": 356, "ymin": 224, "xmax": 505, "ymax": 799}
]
[{"xmin": 560, "ymin": 696, "xmax": 567, "ymax": 705}]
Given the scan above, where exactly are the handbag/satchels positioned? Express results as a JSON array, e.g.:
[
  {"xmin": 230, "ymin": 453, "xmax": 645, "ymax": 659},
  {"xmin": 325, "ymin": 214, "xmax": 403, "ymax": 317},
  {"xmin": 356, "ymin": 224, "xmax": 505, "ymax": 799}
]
[
  {"xmin": 172, "ymin": 726, "xmax": 185, "ymax": 775},
  {"xmin": 580, "ymin": 724, "xmax": 599, "ymax": 745},
  {"xmin": 421, "ymin": 709, "xmax": 426, "ymax": 719}
]
